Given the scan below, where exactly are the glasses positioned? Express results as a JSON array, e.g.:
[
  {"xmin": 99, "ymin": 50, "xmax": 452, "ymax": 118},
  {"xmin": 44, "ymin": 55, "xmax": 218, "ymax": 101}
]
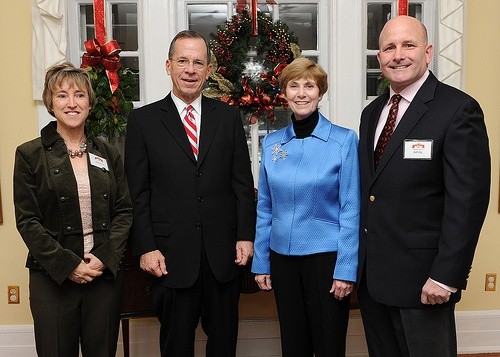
[{"xmin": 170, "ymin": 57, "xmax": 209, "ymax": 68}]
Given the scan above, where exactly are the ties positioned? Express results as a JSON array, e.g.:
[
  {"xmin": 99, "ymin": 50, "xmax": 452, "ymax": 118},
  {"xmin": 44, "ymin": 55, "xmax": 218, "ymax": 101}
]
[
  {"xmin": 374, "ymin": 95, "xmax": 402, "ymax": 172},
  {"xmin": 183, "ymin": 105, "xmax": 198, "ymax": 162}
]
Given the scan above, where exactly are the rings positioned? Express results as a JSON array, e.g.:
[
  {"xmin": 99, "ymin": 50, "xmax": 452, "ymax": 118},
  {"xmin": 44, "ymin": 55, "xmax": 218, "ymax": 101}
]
[
  {"xmin": 80, "ymin": 280, "xmax": 85, "ymax": 284},
  {"xmin": 346, "ymin": 291, "xmax": 351, "ymax": 296}
]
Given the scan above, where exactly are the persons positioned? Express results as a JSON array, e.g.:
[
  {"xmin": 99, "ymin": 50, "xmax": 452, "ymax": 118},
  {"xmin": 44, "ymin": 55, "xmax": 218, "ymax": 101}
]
[
  {"xmin": 125, "ymin": 31, "xmax": 256, "ymax": 357},
  {"xmin": 357, "ymin": 14, "xmax": 491, "ymax": 357},
  {"xmin": 251, "ymin": 57, "xmax": 362, "ymax": 357},
  {"xmin": 13, "ymin": 63, "xmax": 134, "ymax": 357}
]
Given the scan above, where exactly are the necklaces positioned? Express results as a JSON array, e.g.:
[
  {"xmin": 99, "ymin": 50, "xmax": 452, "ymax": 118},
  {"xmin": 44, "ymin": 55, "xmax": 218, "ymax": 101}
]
[{"xmin": 67, "ymin": 139, "xmax": 88, "ymax": 158}]
